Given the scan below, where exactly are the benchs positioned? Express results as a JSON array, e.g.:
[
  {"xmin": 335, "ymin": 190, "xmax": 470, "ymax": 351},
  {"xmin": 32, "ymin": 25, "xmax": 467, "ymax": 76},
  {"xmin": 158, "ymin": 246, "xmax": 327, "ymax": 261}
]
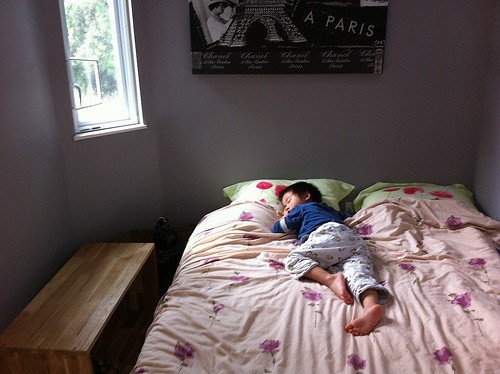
[{"xmin": 0, "ymin": 241, "xmax": 161, "ymax": 374}]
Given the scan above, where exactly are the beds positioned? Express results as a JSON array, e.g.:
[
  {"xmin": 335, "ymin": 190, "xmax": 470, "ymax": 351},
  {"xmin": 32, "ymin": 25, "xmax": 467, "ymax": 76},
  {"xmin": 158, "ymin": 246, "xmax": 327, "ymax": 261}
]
[{"xmin": 130, "ymin": 179, "xmax": 500, "ymax": 374}]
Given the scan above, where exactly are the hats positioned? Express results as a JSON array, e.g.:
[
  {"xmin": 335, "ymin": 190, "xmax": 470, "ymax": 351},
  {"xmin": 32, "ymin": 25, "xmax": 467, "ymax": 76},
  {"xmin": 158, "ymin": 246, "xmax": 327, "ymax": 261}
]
[{"xmin": 207, "ymin": 0, "xmax": 229, "ymax": 9}]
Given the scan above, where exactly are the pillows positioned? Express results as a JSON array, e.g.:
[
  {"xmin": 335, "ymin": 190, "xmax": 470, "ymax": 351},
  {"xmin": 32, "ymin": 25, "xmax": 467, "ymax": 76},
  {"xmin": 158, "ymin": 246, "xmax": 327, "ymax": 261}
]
[
  {"xmin": 354, "ymin": 180, "xmax": 480, "ymax": 215},
  {"xmin": 223, "ymin": 178, "xmax": 356, "ymax": 211}
]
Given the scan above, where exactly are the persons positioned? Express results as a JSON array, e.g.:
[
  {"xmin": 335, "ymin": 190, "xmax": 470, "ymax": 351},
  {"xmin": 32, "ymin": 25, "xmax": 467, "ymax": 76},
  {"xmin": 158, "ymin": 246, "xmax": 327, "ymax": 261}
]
[
  {"xmin": 270, "ymin": 180, "xmax": 392, "ymax": 338},
  {"xmin": 205, "ymin": 1, "xmax": 237, "ymax": 44}
]
